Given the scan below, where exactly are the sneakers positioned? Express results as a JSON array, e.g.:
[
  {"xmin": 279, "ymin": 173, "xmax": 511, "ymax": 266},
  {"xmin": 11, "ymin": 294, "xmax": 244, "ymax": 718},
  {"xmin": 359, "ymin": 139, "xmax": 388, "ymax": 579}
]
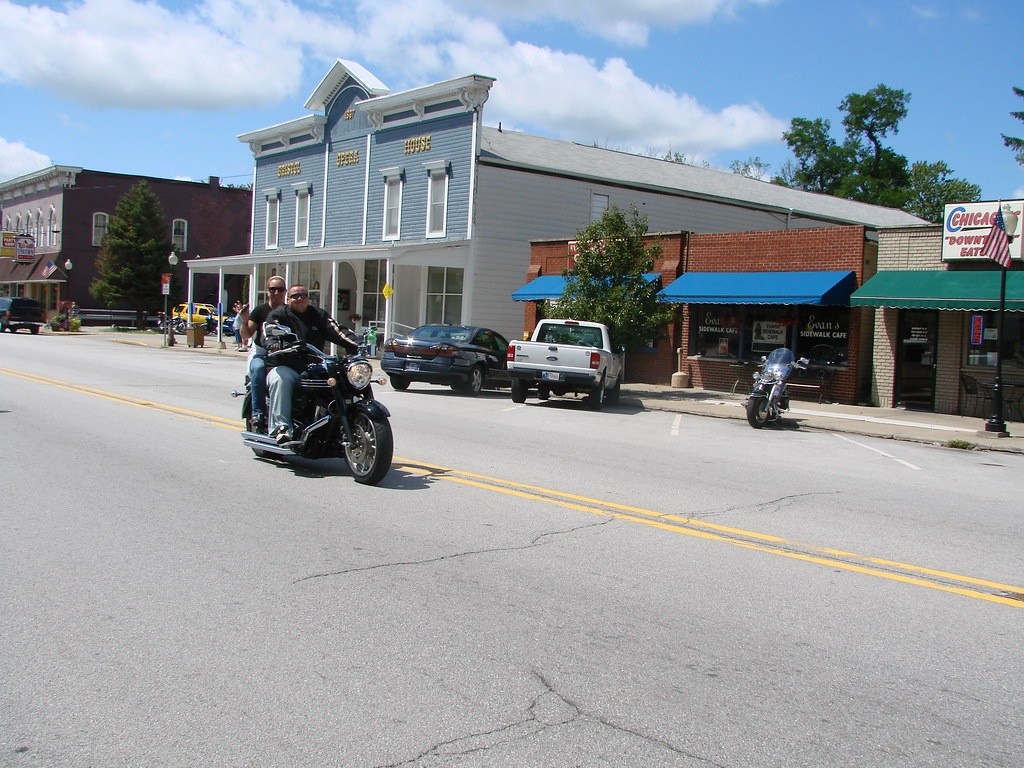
[{"xmin": 276, "ymin": 425, "xmax": 291, "ymax": 444}]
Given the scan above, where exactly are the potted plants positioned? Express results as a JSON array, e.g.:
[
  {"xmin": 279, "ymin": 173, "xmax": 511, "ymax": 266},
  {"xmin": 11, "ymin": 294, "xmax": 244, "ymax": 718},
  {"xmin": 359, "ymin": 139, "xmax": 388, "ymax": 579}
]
[
  {"xmin": 68, "ymin": 316, "xmax": 81, "ymax": 332},
  {"xmin": 367, "ymin": 329, "xmax": 378, "ymax": 356},
  {"xmin": 50, "ymin": 319, "xmax": 60, "ymax": 331},
  {"xmin": 185, "ymin": 323, "xmax": 204, "ymax": 349}
]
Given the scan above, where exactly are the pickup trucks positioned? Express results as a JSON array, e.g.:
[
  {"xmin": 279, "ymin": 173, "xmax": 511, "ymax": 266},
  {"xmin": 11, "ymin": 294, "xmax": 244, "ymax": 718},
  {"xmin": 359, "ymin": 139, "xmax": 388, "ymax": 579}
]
[{"xmin": 505, "ymin": 318, "xmax": 622, "ymax": 412}]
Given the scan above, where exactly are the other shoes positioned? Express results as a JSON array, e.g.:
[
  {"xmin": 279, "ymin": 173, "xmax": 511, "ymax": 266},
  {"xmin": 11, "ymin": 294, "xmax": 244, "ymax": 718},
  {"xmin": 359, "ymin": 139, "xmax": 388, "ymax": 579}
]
[
  {"xmin": 237, "ymin": 348, "xmax": 247, "ymax": 352},
  {"xmin": 252, "ymin": 415, "xmax": 265, "ymax": 426},
  {"xmin": 234, "ymin": 347, "xmax": 239, "ymax": 351}
]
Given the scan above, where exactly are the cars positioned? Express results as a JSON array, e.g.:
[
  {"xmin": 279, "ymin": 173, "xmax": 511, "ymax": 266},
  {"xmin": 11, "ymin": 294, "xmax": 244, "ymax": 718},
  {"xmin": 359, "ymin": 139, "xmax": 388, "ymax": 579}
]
[{"xmin": 378, "ymin": 323, "xmax": 515, "ymax": 395}]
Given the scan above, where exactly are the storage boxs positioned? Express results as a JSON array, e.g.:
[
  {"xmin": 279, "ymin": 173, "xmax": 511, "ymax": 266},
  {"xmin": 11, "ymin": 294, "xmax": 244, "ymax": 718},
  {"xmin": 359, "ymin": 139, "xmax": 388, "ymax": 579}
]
[
  {"xmin": 987, "ymin": 352, "xmax": 997, "ymax": 367},
  {"xmin": 974, "ymin": 355, "xmax": 988, "ymax": 366},
  {"xmin": 969, "ymin": 355, "xmax": 976, "ymax": 365}
]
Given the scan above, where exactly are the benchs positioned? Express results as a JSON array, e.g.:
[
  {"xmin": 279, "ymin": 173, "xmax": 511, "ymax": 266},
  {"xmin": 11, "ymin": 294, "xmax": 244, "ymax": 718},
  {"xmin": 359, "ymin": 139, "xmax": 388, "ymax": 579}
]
[{"xmin": 746, "ymin": 367, "xmax": 836, "ymax": 403}]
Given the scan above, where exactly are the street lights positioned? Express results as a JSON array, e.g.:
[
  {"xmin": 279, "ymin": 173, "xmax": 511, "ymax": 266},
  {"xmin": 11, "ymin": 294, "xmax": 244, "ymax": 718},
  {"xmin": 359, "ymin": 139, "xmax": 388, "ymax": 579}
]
[
  {"xmin": 64, "ymin": 258, "xmax": 72, "ymax": 331},
  {"xmin": 165, "ymin": 251, "xmax": 179, "ymax": 347},
  {"xmin": 983, "ymin": 202, "xmax": 1019, "ymax": 432}
]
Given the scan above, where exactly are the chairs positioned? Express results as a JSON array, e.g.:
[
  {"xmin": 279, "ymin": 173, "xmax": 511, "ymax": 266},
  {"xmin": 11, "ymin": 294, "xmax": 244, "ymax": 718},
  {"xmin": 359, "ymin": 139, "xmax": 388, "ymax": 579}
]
[
  {"xmin": 960, "ymin": 375, "xmax": 992, "ymax": 421},
  {"xmin": 545, "ymin": 330, "xmax": 560, "ymax": 343},
  {"xmin": 1002, "ymin": 388, "xmax": 1024, "ymax": 423},
  {"xmin": 577, "ymin": 333, "xmax": 594, "ymax": 346}
]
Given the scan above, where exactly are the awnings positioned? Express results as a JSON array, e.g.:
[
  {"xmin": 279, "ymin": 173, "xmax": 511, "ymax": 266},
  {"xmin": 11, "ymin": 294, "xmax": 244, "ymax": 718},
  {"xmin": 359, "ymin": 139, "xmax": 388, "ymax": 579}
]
[
  {"xmin": 656, "ymin": 270, "xmax": 855, "ymax": 306},
  {"xmin": 512, "ymin": 272, "xmax": 660, "ymax": 301},
  {"xmin": 0, "ymin": 253, "xmax": 66, "ymax": 282},
  {"xmin": 850, "ymin": 270, "xmax": 1024, "ymax": 313}
]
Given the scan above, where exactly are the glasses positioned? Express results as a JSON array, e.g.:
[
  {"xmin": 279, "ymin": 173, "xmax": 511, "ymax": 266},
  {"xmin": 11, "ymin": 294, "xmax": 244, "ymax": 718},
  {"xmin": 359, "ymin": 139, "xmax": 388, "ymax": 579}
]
[
  {"xmin": 267, "ymin": 286, "xmax": 285, "ymax": 293},
  {"xmin": 289, "ymin": 292, "xmax": 308, "ymax": 299}
]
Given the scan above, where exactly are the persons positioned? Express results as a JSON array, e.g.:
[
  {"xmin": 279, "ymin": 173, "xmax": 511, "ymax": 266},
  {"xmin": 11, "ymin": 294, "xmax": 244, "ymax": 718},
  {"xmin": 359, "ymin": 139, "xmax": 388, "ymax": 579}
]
[
  {"xmin": 260, "ymin": 284, "xmax": 370, "ymax": 444},
  {"xmin": 233, "ymin": 300, "xmax": 242, "ymax": 351},
  {"xmin": 233, "ymin": 276, "xmax": 287, "ymax": 431}
]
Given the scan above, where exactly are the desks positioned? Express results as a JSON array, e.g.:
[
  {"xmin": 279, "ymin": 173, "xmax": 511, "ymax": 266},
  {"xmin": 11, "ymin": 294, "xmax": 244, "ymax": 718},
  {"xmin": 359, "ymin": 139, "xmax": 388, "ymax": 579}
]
[{"xmin": 982, "ymin": 383, "xmax": 1024, "ymax": 388}]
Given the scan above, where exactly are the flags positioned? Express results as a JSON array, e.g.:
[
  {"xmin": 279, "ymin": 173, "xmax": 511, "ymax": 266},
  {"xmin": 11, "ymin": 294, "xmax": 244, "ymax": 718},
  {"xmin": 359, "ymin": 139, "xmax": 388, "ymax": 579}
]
[
  {"xmin": 382, "ymin": 283, "xmax": 393, "ymax": 299},
  {"xmin": 981, "ymin": 208, "xmax": 1012, "ymax": 269},
  {"xmin": 40, "ymin": 260, "xmax": 57, "ymax": 278}
]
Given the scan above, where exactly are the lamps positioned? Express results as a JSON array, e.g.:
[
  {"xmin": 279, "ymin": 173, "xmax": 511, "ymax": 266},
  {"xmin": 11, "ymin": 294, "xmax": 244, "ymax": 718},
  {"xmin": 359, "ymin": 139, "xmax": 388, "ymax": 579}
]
[
  {"xmin": 364, "ymin": 274, "xmax": 368, "ymax": 282},
  {"xmin": 52, "ymin": 231, "xmax": 60, "ymax": 234},
  {"xmin": 195, "ymin": 255, "xmax": 201, "ymax": 260}
]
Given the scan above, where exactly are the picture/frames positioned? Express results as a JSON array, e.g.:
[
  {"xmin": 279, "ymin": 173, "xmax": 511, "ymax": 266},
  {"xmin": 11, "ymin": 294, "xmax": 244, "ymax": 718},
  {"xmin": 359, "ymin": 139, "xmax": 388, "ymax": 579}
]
[
  {"xmin": 337, "ymin": 289, "xmax": 350, "ymax": 311},
  {"xmin": 272, "ymin": 268, "xmax": 276, "ymax": 276}
]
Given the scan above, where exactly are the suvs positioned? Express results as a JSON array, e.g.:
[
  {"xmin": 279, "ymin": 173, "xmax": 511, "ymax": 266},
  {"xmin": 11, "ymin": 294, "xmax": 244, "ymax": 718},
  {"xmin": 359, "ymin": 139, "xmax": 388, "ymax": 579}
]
[
  {"xmin": 0, "ymin": 296, "xmax": 47, "ymax": 335},
  {"xmin": 170, "ymin": 302, "xmax": 229, "ymax": 329}
]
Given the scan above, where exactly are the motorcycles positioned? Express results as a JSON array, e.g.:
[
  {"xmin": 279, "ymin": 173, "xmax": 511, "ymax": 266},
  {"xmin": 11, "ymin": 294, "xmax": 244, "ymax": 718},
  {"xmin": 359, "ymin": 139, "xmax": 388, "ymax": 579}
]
[
  {"xmin": 741, "ymin": 348, "xmax": 810, "ymax": 430},
  {"xmin": 156, "ymin": 310, "xmax": 236, "ymax": 335},
  {"xmin": 231, "ymin": 324, "xmax": 394, "ymax": 485}
]
